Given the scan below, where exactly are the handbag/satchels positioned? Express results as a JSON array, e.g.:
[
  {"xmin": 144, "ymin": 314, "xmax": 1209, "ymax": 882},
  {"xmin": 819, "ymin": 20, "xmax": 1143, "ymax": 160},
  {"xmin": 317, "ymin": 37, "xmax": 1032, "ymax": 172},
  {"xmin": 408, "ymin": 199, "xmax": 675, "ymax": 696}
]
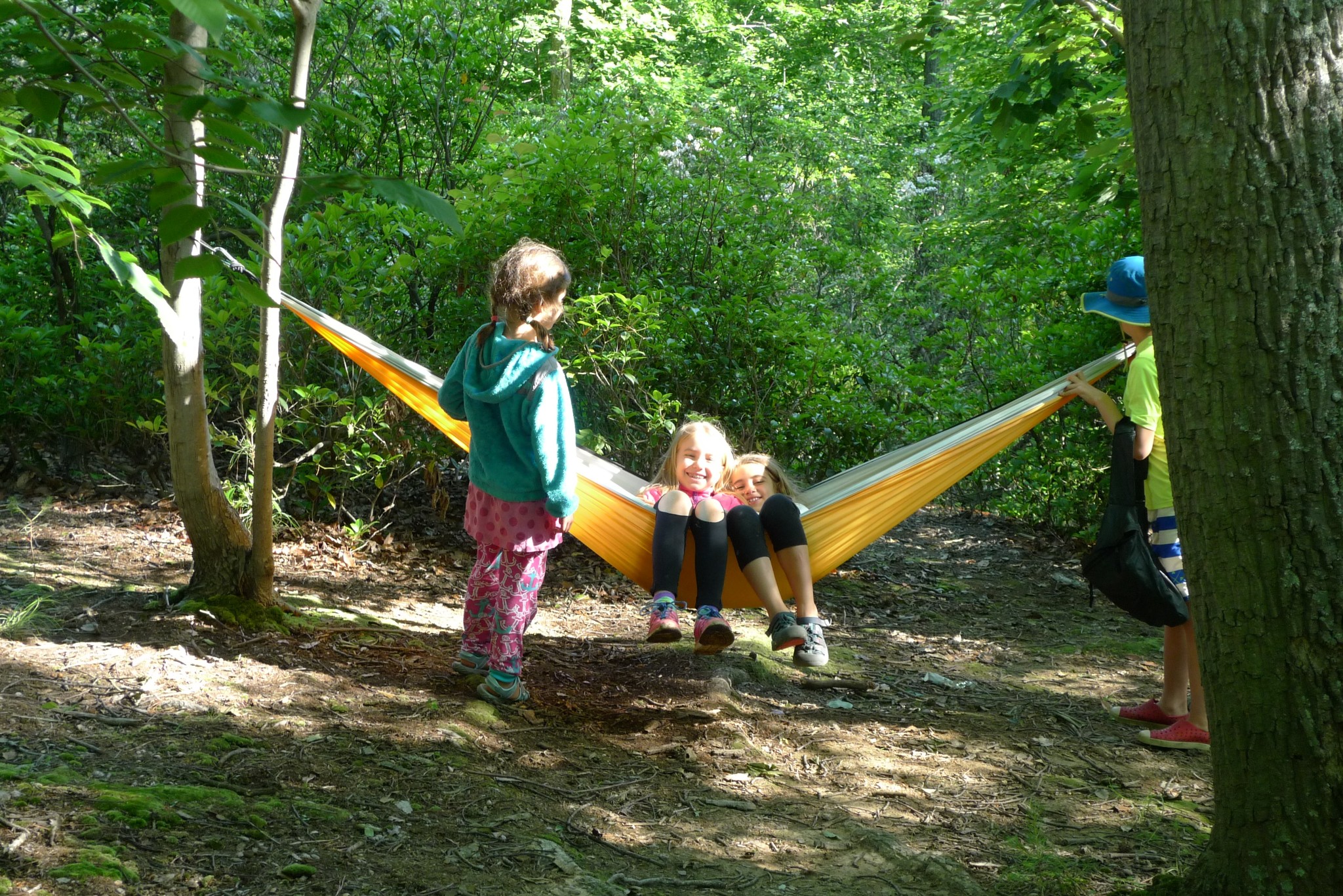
[{"xmin": 1079, "ymin": 416, "xmax": 1190, "ymax": 629}]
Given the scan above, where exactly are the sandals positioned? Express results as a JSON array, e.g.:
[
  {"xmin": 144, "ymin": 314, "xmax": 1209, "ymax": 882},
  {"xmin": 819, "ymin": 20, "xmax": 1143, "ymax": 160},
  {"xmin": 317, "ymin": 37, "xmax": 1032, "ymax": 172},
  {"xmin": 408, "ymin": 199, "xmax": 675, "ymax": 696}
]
[
  {"xmin": 451, "ymin": 651, "xmax": 490, "ymax": 677},
  {"xmin": 1138, "ymin": 719, "xmax": 1211, "ymax": 752},
  {"xmin": 477, "ymin": 675, "xmax": 530, "ymax": 705},
  {"xmin": 1110, "ymin": 697, "xmax": 1191, "ymax": 729}
]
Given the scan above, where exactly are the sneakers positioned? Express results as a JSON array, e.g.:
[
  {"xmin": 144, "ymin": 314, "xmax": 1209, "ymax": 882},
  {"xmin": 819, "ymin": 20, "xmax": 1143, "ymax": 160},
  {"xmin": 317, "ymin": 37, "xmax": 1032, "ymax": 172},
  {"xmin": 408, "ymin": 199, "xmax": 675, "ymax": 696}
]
[
  {"xmin": 793, "ymin": 616, "xmax": 831, "ymax": 668},
  {"xmin": 765, "ymin": 612, "xmax": 807, "ymax": 651},
  {"xmin": 694, "ymin": 605, "xmax": 734, "ymax": 656},
  {"xmin": 639, "ymin": 601, "xmax": 687, "ymax": 643}
]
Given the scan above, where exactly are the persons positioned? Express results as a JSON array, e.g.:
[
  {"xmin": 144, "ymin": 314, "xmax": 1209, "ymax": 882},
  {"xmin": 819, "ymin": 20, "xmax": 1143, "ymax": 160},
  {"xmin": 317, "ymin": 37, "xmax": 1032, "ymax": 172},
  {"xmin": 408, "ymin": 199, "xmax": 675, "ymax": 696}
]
[
  {"xmin": 1058, "ymin": 256, "xmax": 1213, "ymax": 750},
  {"xmin": 438, "ymin": 235, "xmax": 579, "ymax": 709},
  {"xmin": 638, "ymin": 421, "xmax": 747, "ymax": 655},
  {"xmin": 726, "ymin": 452, "xmax": 829, "ymax": 667}
]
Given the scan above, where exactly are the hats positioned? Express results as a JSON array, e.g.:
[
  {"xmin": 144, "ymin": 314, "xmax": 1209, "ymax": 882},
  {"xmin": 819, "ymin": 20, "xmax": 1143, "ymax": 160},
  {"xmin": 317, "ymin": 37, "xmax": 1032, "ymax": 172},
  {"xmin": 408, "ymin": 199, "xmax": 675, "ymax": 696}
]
[{"xmin": 1080, "ymin": 255, "xmax": 1151, "ymax": 326}]
